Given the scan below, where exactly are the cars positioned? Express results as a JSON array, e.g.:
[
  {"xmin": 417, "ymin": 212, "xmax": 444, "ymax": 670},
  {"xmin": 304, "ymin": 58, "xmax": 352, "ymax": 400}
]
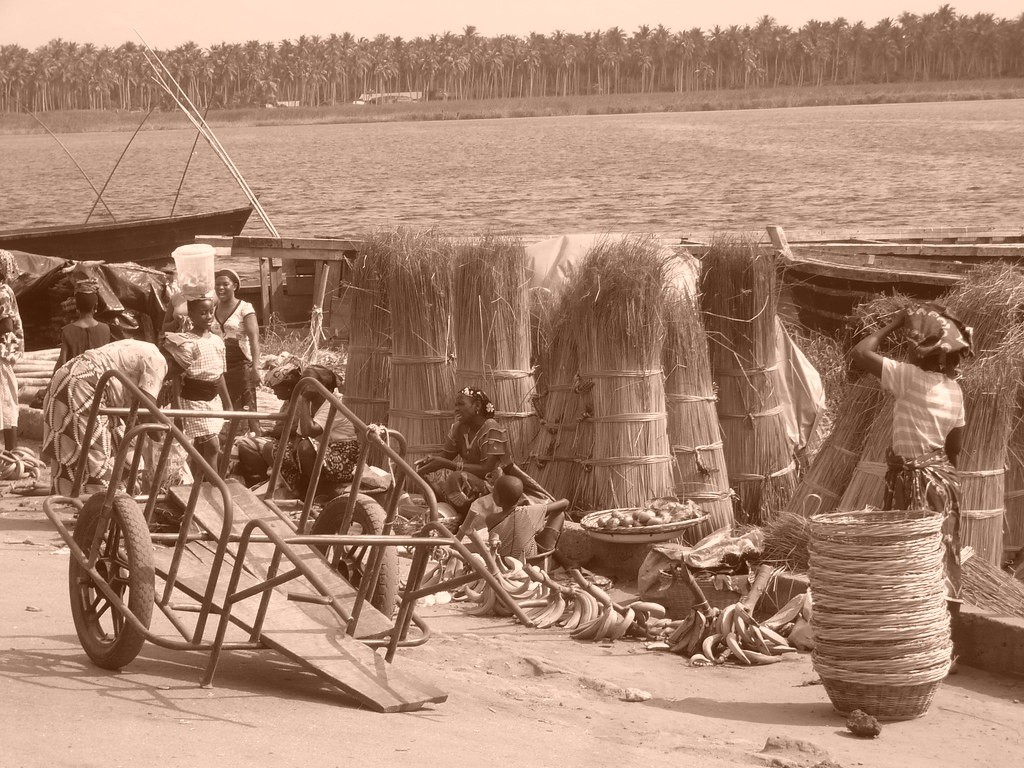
[{"xmin": 259, "ymin": 91, "xmax": 425, "ymax": 111}]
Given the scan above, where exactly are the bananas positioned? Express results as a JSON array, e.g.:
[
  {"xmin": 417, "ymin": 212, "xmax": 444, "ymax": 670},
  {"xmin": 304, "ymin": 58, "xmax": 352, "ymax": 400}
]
[{"xmin": 418, "ymin": 549, "xmax": 796, "ymax": 665}]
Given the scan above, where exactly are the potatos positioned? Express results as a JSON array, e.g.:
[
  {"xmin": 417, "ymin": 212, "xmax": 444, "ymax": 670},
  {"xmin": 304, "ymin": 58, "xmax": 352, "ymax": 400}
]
[{"xmin": 597, "ymin": 510, "xmax": 664, "ymax": 529}]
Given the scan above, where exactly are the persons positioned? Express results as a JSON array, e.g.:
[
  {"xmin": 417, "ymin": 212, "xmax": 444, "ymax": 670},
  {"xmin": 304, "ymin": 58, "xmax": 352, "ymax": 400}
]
[
  {"xmin": 236, "ymin": 362, "xmax": 300, "ymax": 486},
  {"xmin": 158, "ymin": 268, "xmax": 261, "ymax": 481},
  {"xmin": 52, "ymin": 283, "xmax": 110, "ymax": 376},
  {"xmin": 109, "ymin": 307, "xmax": 156, "ymax": 344},
  {"xmin": 0, "ymin": 249, "xmax": 23, "ymax": 451},
  {"xmin": 413, "ymin": 385, "xmax": 508, "ymax": 514},
  {"xmin": 264, "ymin": 364, "xmax": 359, "ymax": 508},
  {"xmin": 484, "ymin": 477, "xmax": 571, "ymax": 574},
  {"xmin": 43, "ymin": 336, "xmax": 199, "ymax": 504},
  {"xmin": 850, "ymin": 302, "xmax": 974, "ymax": 673}
]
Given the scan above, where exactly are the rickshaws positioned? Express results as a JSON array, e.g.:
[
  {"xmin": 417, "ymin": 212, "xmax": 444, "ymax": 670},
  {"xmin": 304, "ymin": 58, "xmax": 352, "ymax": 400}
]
[{"xmin": 43, "ymin": 370, "xmax": 534, "ymax": 715}]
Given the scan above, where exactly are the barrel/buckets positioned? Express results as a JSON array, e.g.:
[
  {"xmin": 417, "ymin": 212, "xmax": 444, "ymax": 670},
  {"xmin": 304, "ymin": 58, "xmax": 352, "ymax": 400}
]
[{"xmin": 170, "ymin": 244, "xmax": 215, "ymax": 298}]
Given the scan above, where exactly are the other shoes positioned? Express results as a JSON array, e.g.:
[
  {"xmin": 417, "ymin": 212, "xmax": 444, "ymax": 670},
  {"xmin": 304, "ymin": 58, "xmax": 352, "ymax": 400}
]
[{"xmin": 248, "ymin": 471, "xmax": 270, "ymax": 491}]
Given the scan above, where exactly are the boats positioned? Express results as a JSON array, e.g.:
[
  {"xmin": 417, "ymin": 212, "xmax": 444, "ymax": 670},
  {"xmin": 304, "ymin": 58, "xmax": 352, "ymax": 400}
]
[
  {"xmin": 0, "ymin": 194, "xmax": 259, "ymax": 267},
  {"xmin": 727, "ymin": 241, "xmax": 1024, "ymax": 342}
]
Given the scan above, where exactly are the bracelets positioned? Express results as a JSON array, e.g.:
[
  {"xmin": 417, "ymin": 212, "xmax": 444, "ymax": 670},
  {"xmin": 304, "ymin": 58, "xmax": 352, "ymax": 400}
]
[{"xmin": 456, "ymin": 461, "xmax": 464, "ymax": 471}]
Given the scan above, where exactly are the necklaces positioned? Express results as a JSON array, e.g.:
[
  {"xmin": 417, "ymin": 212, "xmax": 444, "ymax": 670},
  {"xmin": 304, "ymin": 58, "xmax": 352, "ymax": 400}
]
[{"xmin": 463, "ymin": 426, "xmax": 482, "ymax": 454}]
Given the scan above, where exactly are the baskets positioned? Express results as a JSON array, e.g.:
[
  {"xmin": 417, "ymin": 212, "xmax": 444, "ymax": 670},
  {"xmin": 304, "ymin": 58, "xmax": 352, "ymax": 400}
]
[
  {"xmin": 581, "ymin": 508, "xmax": 711, "ymax": 534},
  {"xmin": 806, "ymin": 509, "xmax": 953, "ymax": 722}
]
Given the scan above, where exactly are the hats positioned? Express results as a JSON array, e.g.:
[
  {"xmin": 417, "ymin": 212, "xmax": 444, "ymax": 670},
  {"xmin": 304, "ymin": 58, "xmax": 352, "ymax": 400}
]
[
  {"xmin": 214, "ymin": 268, "xmax": 241, "ymax": 290},
  {"xmin": 162, "ymin": 332, "xmax": 194, "ymax": 370}
]
